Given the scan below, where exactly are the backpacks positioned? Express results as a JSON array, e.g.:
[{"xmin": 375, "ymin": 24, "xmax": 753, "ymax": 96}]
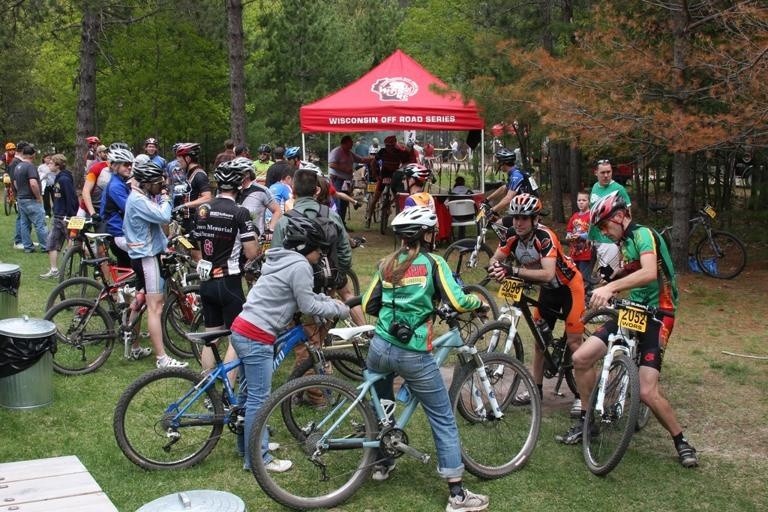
[{"xmin": 286, "ymin": 204, "xmax": 338, "ymax": 289}]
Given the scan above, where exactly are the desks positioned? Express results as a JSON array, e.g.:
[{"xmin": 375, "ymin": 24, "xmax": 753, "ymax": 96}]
[{"xmin": 0, "ymin": 454, "xmax": 120, "ymax": 512}]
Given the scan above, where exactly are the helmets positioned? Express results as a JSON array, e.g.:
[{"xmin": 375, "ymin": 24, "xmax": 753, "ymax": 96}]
[
  {"xmin": 590, "ymin": 190, "xmax": 626, "ymax": 225},
  {"xmin": 391, "ymin": 206, "xmax": 438, "ymax": 240},
  {"xmin": 107, "ymin": 138, "xmax": 200, "ymax": 184},
  {"xmin": 85, "ymin": 137, "xmax": 99, "ymax": 144},
  {"xmin": 509, "ymin": 194, "xmax": 542, "ymax": 216},
  {"xmin": 384, "ymin": 136, "xmax": 397, "ymax": 145},
  {"xmin": 496, "ymin": 149, "xmax": 515, "ymax": 164},
  {"xmin": 5, "ymin": 143, "xmax": 16, "ymax": 150},
  {"xmin": 258, "ymin": 145, "xmax": 271, "ymax": 153},
  {"xmin": 396, "ymin": 163, "xmax": 429, "ymax": 183},
  {"xmin": 214, "ymin": 157, "xmax": 255, "ymax": 191},
  {"xmin": 285, "ymin": 147, "xmax": 301, "ymax": 159},
  {"xmin": 283, "ymin": 217, "xmax": 332, "ymax": 246}
]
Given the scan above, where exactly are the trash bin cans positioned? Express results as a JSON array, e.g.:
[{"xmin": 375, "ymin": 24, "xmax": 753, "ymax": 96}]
[
  {"xmin": 0, "ymin": 315, "xmax": 57, "ymax": 409},
  {"xmin": 134, "ymin": 490, "xmax": 248, "ymax": 512},
  {"xmin": 0, "ymin": 264, "xmax": 23, "ymax": 319}
]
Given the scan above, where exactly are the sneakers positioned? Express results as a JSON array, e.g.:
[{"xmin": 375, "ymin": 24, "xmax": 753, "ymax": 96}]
[
  {"xmin": 354, "ymin": 200, "xmax": 362, "ymax": 209},
  {"xmin": 32, "ymin": 242, "xmax": 39, "ymax": 247},
  {"xmin": 571, "ymin": 399, "xmax": 582, "ymax": 416},
  {"xmin": 512, "ymin": 389, "xmax": 543, "ymax": 405},
  {"xmin": 555, "ymin": 423, "xmax": 592, "ymax": 445},
  {"xmin": 124, "ymin": 284, "xmax": 145, "ymax": 297},
  {"xmin": 267, "ymin": 443, "xmax": 279, "ymax": 450},
  {"xmin": 156, "ymin": 356, "xmax": 189, "ymax": 368},
  {"xmin": 41, "ymin": 271, "xmax": 60, "ymax": 280},
  {"xmin": 372, "ymin": 464, "xmax": 395, "ymax": 480},
  {"xmin": 14, "ymin": 244, "xmax": 27, "ymax": 249},
  {"xmin": 129, "ymin": 347, "xmax": 152, "ymax": 360},
  {"xmin": 261, "ymin": 458, "xmax": 292, "ymax": 473},
  {"xmin": 676, "ymin": 443, "xmax": 697, "ymax": 466},
  {"xmin": 446, "ymin": 489, "xmax": 490, "ymax": 512}
]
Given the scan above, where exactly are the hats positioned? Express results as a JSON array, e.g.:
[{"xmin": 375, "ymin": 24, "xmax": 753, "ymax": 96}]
[{"xmin": 96, "ymin": 145, "xmax": 108, "ymax": 153}]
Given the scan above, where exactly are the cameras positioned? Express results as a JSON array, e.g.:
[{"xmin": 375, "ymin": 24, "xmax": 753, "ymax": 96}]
[{"xmin": 388, "ymin": 320, "xmax": 413, "ymax": 343}]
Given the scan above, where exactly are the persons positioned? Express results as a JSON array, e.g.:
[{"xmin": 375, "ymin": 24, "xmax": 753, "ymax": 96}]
[{"xmin": 1, "ymin": 135, "xmax": 698, "ymax": 512}]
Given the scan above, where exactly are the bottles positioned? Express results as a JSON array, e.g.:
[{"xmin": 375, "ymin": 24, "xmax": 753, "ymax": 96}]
[
  {"xmin": 397, "ymin": 380, "xmax": 413, "ymax": 406},
  {"xmin": 89, "ymin": 240, "xmax": 97, "ymax": 255},
  {"xmin": 688, "ymin": 253, "xmax": 718, "ymax": 274},
  {"xmin": 536, "ymin": 318, "xmax": 553, "ymax": 346},
  {"xmin": 131, "ymin": 291, "xmax": 145, "ymax": 311}
]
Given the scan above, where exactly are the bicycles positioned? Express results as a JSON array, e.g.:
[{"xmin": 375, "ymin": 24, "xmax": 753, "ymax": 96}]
[
  {"xmin": 0, "ymin": 173, "xmax": 20, "ymax": 216},
  {"xmin": 245, "ymin": 301, "xmax": 543, "ymax": 512},
  {"xmin": 650, "ymin": 202, "xmax": 748, "ymax": 280},
  {"xmin": 42, "ymin": 164, "xmax": 676, "ymax": 477}
]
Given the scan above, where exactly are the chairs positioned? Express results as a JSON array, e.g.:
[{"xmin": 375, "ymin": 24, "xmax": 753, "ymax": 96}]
[{"xmin": 446, "ymin": 200, "xmax": 484, "ymax": 245}]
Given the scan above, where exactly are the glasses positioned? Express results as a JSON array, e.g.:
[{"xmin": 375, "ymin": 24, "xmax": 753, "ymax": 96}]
[{"xmin": 598, "ymin": 160, "xmax": 610, "ymax": 163}]
